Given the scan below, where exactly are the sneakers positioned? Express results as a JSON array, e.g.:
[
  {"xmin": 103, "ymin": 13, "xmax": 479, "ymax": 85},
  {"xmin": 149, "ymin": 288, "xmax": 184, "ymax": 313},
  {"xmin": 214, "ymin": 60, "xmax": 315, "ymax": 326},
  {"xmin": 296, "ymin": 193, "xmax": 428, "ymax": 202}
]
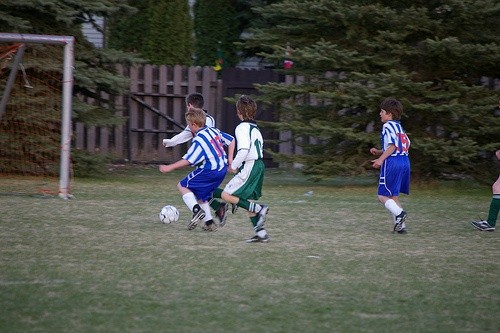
[
  {"xmin": 232, "ymin": 204, "xmax": 238, "ymax": 214},
  {"xmin": 202, "ymin": 223, "xmax": 219, "ymax": 232},
  {"xmin": 257, "ymin": 204, "xmax": 270, "ymax": 228},
  {"xmin": 214, "ymin": 203, "xmax": 228, "ymax": 227},
  {"xmin": 471, "ymin": 216, "xmax": 495, "ymax": 232},
  {"xmin": 187, "ymin": 206, "xmax": 206, "ymax": 230},
  {"xmin": 393, "ymin": 211, "xmax": 408, "ymax": 231},
  {"xmin": 394, "ymin": 228, "xmax": 408, "ymax": 234},
  {"xmin": 245, "ymin": 234, "xmax": 269, "ymax": 243}
]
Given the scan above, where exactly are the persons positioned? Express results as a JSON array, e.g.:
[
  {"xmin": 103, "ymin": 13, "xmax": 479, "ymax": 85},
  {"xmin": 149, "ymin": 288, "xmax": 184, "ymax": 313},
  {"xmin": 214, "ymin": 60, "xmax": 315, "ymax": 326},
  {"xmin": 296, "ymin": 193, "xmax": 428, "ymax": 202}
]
[
  {"xmin": 471, "ymin": 150, "xmax": 500, "ymax": 232},
  {"xmin": 220, "ymin": 95, "xmax": 269, "ymax": 243},
  {"xmin": 370, "ymin": 99, "xmax": 410, "ymax": 233},
  {"xmin": 159, "ymin": 92, "xmax": 238, "ymax": 233}
]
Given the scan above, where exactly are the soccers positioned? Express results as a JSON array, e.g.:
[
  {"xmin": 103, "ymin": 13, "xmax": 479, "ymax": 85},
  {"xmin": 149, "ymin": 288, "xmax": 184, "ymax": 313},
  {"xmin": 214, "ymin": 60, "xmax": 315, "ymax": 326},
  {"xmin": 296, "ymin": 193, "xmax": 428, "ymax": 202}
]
[{"xmin": 159, "ymin": 204, "xmax": 179, "ymax": 225}]
